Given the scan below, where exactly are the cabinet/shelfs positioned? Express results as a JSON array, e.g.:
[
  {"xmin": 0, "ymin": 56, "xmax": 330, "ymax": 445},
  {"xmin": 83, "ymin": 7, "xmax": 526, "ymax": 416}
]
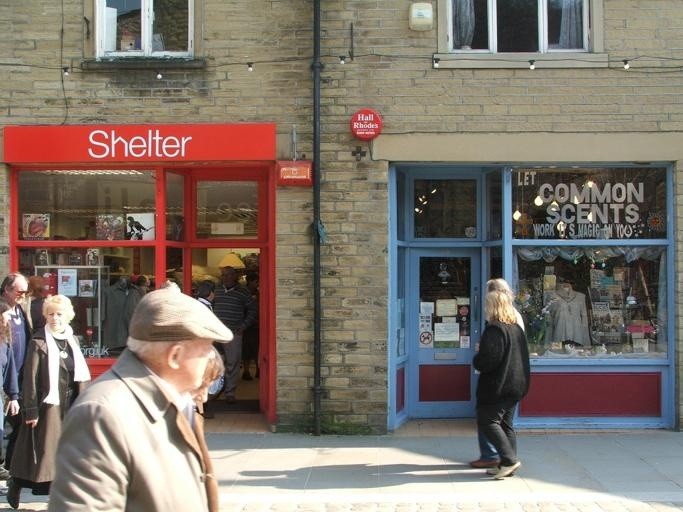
[
  {"xmin": 82, "ymin": 251, "xmax": 132, "ymax": 278},
  {"xmin": 30, "ymin": 263, "xmax": 109, "ymax": 358}
]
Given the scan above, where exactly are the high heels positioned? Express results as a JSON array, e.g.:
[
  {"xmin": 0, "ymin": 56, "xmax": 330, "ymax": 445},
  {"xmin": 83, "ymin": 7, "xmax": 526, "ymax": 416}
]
[{"xmin": 6, "ymin": 476, "xmax": 21, "ymax": 508}]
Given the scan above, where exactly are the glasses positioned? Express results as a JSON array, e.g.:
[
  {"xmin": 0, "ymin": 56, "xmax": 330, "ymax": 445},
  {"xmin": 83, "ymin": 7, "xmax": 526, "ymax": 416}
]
[{"xmin": 14, "ymin": 290, "xmax": 29, "ymax": 297}]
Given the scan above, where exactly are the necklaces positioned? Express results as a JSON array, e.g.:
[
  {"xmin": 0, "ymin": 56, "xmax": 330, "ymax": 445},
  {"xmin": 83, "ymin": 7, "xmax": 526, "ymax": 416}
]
[
  {"xmin": 52, "ymin": 338, "xmax": 68, "ymax": 358},
  {"xmin": 556, "ymin": 292, "xmax": 577, "ymax": 303}
]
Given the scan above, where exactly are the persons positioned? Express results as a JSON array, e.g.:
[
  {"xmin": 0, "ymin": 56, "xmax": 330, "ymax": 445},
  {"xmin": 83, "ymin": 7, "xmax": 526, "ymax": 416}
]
[
  {"xmin": 127, "ymin": 216, "xmax": 148, "ymax": 241},
  {"xmin": 473, "ymin": 291, "xmax": 532, "ymax": 482},
  {"xmin": 541, "ymin": 282, "xmax": 591, "ymax": 350},
  {"xmin": 174, "ymin": 347, "xmax": 219, "ymax": 511},
  {"xmin": 0, "ymin": 272, "xmax": 33, "ymax": 469},
  {"xmin": 196, "ymin": 280, "xmax": 225, "ymax": 419},
  {"xmin": 465, "ymin": 277, "xmax": 526, "ymax": 468},
  {"xmin": 0, "ymin": 293, "xmax": 20, "ymax": 480},
  {"xmin": 5, "ymin": 292, "xmax": 91, "ymax": 509},
  {"xmin": 46, "ymin": 286, "xmax": 235, "ymax": 512},
  {"xmin": 240, "ymin": 271, "xmax": 258, "ymax": 383},
  {"xmin": 210, "ymin": 265, "xmax": 258, "ymax": 404}
]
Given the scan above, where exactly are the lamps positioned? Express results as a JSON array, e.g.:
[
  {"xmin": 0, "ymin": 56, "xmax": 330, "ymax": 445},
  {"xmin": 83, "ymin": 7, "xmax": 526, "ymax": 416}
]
[{"xmin": 507, "ymin": 169, "xmax": 602, "ymax": 233}]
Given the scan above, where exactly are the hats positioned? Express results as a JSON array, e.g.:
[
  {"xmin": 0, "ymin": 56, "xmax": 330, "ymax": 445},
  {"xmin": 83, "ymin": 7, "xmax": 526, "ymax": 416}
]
[{"xmin": 127, "ymin": 287, "xmax": 235, "ymax": 343}]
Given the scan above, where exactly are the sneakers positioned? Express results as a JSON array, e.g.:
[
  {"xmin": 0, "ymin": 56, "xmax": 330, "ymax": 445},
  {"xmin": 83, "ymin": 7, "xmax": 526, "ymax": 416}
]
[
  {"xmin": 226, "ymin": 392, "xmax": 236, "ymax": 405},
  {"xmin": 0, "ymin": 466, "xmax": 10, "ymax": 496},
  {"xmin": 493, "ymin": 460, "xmax": 521, "ymax": 480}
]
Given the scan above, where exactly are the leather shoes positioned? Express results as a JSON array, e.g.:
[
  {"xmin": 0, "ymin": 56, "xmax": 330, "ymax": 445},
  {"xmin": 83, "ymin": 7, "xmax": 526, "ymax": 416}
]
[
  {"xmin": 469, "ymin": 457, "xmax": 501, "ymax": 468},
  {"xmin": 485, "ymin": 469, "xmax": 514, "ymax": 477}
]
